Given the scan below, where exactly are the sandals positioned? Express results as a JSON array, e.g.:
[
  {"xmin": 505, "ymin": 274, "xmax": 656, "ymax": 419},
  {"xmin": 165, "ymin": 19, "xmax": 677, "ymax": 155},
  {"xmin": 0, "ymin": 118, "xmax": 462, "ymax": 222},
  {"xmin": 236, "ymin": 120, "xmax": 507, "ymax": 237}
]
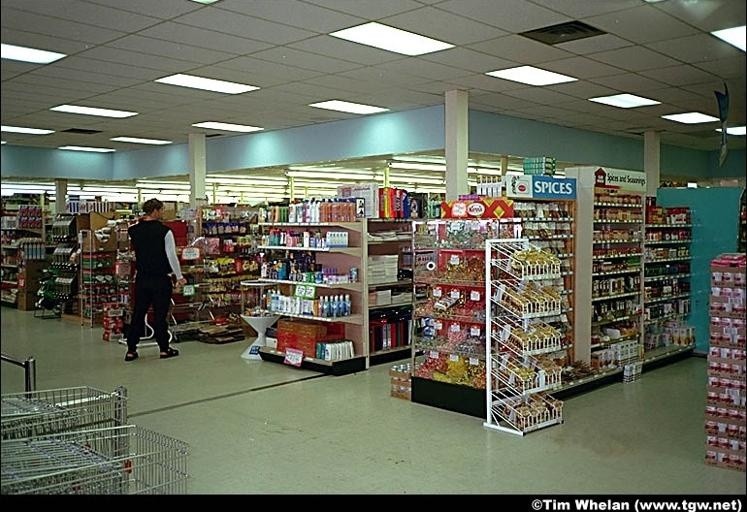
[
  {"xmin": 124, "ymin": 350, "xmax": 139, "ymax": 361},
  {"xmin": 159, "ymin": 347, "xmax": 179, "ymax": 359}
]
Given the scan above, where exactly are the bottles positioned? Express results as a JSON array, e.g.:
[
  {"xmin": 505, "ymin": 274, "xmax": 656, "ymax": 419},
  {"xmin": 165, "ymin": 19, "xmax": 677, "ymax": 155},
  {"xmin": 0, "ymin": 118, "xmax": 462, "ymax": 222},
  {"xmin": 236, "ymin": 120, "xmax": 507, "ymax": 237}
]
[
  {"xmin": 260, "ymin": 227, "xmax": 327, "ymax": 248},
  {"xmin": 260, "ymin": 251, "xmax": 352, "ymax": 320},
  {"xmin": 391, "ymin": 363, "xmax": 419, "ymax": 393},
  {"xmin": 267, "ymin": 196, "xmax": 357, "ymax": 223}
]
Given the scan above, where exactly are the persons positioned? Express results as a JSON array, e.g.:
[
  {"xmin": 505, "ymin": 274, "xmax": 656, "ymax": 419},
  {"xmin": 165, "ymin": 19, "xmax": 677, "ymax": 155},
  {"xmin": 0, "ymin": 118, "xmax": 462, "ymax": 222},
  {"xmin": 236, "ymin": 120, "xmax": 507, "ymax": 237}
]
[{"xmin": 122, "ymin": 197, "xmax": 191, "ymax": 362}]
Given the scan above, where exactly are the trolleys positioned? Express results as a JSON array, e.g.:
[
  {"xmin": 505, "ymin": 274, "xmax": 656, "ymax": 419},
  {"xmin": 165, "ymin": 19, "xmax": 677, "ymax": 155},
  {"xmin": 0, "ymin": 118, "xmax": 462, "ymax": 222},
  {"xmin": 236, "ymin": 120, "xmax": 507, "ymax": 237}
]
[{"xmin": 1, "ymin": 350, "xmax": 197, "ymax": 495}]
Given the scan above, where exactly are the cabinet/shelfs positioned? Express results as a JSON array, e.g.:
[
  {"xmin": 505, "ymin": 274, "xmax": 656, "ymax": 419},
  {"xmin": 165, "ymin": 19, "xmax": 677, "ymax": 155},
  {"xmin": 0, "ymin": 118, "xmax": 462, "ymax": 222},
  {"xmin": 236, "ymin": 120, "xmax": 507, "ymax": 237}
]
[{"xmin": 591, "ymin": 194, "xmax": 696, "ymax": 391}]
